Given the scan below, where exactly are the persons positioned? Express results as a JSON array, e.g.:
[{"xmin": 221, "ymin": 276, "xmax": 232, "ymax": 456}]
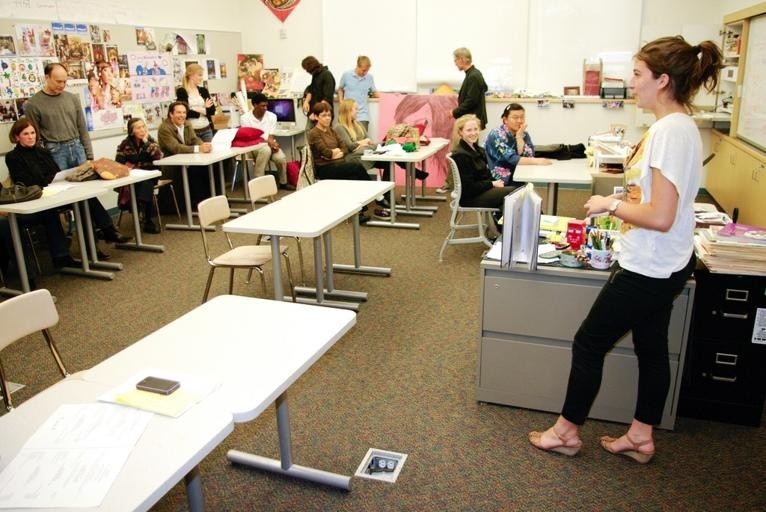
[
  {"xmin": 483, "ymin": 103, "xmax": 553, "ymax": 187},
  {"xmin": 301, "ymin": 56, "xmax": 336, "ymax": 144},
  {"xmin": 435, "ymin": 48, "xmax": 488, "ymax": 194},
  {"xmin": 239, "ymin": 58, "xmax": 262, "ymax": 102},
  {"xmin": 240, "ymin": 94, "xmax": 296, "ymax": 191},
  {"xmin": 114, "ymin": 63, "xmax": 216, "ymax": 234},
  {"xmin": 453, "ymin": 114, "xmax": 517, "ymax": 241},
  {"xmin": 308, "ymin": 103, "xmax": 390, "ymax": 223},
  {"xmin": 527, "ymin": 37, "xmax": 725, "ymax": 465},
  {"xmin": 0, "ymin": 63, "xmax": 135, "ymax": 303},
  {"xmin": 337, "ymin": 56, "xmax": 380, "ymax": 133},
  {"xmin": 338, "ymin": 99, "xmax": 429, "ymax": 203}
]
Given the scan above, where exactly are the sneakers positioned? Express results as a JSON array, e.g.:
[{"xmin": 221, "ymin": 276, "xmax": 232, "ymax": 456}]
[
  {"xmin": 435, "ymin": 186, "xmax": 451, "ymax": 193},
  {"xmin": 280, "ymin": 183, "xmax": 296, "ymax": 191},
  {"xmin": 0, "ymin": 184, "xmax": 42, "ymax": 204},
  {"xmin": 144, "ymin": 223, "xmax": 160, "ymax": 234}
]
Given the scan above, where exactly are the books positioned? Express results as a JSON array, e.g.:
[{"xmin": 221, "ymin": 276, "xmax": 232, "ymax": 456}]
[
  {"xmin": 500, "ymin": 183, "xmax": 543, "ymax": 271},
  {"xmin": 695, "ymin": 222, "xmax": 766, "ymax": 276}
]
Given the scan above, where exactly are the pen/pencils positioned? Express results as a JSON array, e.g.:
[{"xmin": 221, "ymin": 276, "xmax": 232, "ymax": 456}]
[{"xmin": 590, "ymin": 222, "xmax": 616, "ymax": 250}]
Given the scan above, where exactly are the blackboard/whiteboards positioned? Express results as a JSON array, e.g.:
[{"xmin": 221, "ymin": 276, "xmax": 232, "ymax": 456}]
[
  {"xmin": 0, "ymin": 17, "xmax": 242, "ymax": 157},
  {"xmin": 736, "ymin": 13, "xmax": 766, "ymax": 154}
]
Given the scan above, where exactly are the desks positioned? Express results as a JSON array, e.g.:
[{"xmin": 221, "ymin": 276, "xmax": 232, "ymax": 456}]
[
  {"xmin": 360, "ymin": 136, "xmax": 450, "ymax": 230},
  {"xmin": 270, "ymin": 129, "xmax": 305, "ymax": 162},
  {"xmin": 222, "ymin": 179, "xmax": 395, "ymax": 310},
  {"xmin": 512, "ymin": 154, "xmax": 593, "ymax": 216},
  {"xmin": 153, "ymin": 143, "xmax": 265, "ymax": 232},
  {"xmin": 0, "ymin": 168, "xmax": 162, "ymax": 305}
]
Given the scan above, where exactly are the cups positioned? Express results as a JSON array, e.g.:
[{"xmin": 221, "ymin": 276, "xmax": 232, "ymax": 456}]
[
  {"xmin": 559, "ymin": 251, "xmax": 583, "ymax": 267},
  {"xmin": 585, "ymin": 249, "xmax": 612, "ymax": 270}
]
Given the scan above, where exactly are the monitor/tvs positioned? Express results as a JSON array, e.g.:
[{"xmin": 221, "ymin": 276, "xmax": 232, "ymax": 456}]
[{"xmin": 266, "ymin": 98, "xmax": 297, "ymax": 129}]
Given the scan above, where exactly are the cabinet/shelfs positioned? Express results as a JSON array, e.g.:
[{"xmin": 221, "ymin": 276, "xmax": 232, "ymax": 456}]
[
  {"xmin": 478, "ymin": 231, "xmax": 696, "ymax": 432},
  {"xmin": 705, "ymin": 2, "xmax": 766, "ymax": 234}
]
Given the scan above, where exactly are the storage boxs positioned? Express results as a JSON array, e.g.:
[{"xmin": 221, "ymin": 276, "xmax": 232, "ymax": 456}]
[
  {"xmin": 583, "ymin": 59, "xmax": 603, "ymax": 96},
  {"xmin": 603, "ymin": 88, "xmax": 624, "ymax": 98},
  {"xmin": 602, "ymin": 76, "xmax": 624, "ymax": 88}
]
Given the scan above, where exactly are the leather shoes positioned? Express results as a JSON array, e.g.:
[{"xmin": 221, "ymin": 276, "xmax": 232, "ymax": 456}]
[
  {"xmin": 87, "ymin": 246, "xmax": 110, "ymax": 261},
  {"xmin": 375, "ymin": 198, "xmax": 390, "ymax": 208},
  {"xmin": 415, "ymin": 168, "xmax": 429, "ymax": 180},
  {"xmin": 359, "ymin": 214, "xmax": 371, "ymax": 224},
  {"xmin": 54, "ymin": 256, "xmax": 79, "ymax": 266},
  {"xmin": 104, "ymin": 231, "xmax": 135, "ymax": 243}
]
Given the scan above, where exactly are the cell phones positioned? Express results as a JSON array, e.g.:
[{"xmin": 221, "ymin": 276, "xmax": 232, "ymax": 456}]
[{"xmin": 136, "ymin": 376, "xmax": 180, "ymax": 394}]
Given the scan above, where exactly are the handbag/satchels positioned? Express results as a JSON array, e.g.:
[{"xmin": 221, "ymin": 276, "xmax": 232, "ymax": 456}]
[
  {"xmin": 534, "ymin": 143, "xmax": 586, "ymax": 160},
  {"xmin": 387, "ymin": 123, "xmax": 420, "ymax": 149},
  {"xmin": 118, "ymin": 186, "xmax": 130, "ymax": 210}
]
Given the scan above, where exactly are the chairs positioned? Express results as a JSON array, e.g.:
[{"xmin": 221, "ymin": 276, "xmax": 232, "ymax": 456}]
[
  {"xmin": 198, "ymin": 195, "xmax": 296, "ymax": 300},
  {"xmin": 247, "ymin": 174, "xmax": 306, "ymax": 284},
  {"xmin": 24, "ymin": 226, "xmax": 54, "ymax": 278},
  {"xmin": 299, "ymin": 144, "xmax": 350, "ymax": 226},
  {"xmin": 230, "ymin": 154, "xmax": 272, "ymax": 190},
  {"xmin": 117, "ymin": 179, "xmax": 182, "ymax": 229},
  {"xmin": 0, "ymin": 288, "xmax": 70, "ymax": 411},
  {"xmin": 438, "ymin": 153, "xmax": 500, "ymax": 261}
]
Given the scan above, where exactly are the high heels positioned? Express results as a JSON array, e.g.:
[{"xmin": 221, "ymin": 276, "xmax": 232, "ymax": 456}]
[
  {"xmin": 601, "ymin": 433, "xmax": 655, "ymax": 464},
  {"xmin": 528, "ymin": 427, "xmax": 582, "ymax": 457}
]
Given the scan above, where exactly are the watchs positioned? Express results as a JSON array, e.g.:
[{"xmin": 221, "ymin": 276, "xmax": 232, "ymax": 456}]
[{"xmin": 609, "ymin": 199, "xmax": 622, "ymax": 216}]
